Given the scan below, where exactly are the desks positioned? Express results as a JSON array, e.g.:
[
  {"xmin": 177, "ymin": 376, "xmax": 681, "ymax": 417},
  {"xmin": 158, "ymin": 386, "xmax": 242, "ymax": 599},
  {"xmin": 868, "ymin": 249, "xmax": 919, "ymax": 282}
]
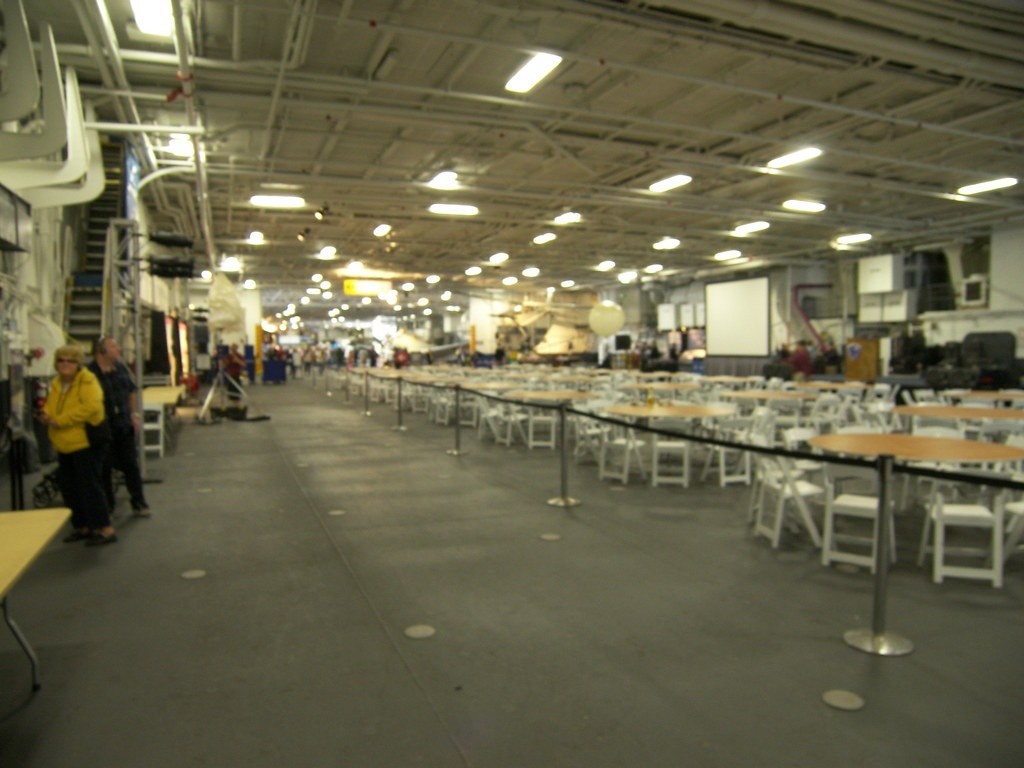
[
  {"xmin": 802, "ymin": 381, "xmax": 874, "ymax": 399},
  {"xmin": 0, "ymin": 506, "xmax": 73, "ymax": 691},
  {"xmin": 890, "ymin": 404, "xmax": 1024, "ymax": 442},
  {"xmin": 381, "ymin": 372, "xmax": 405, "ymax": 380},
  {"xmin": 961, "ymin": 391, "xmax": 1024, "ymax": 407},
  {"xmin": 507, "ymin": 389, "xmax": 596, "ymax": 451},
  {"xmin": 549, "ymin": 374, "xmax": 589, "ymax": 389},
  {"xmin": 639, "ymin": 370, "xmax": 682, "ymax": 382},
  {"xmin": 809, "ymin": 435, "xmax": 1024, "ymax": 574},
  {"xmin": 405, "ymin": 375, "xmax": 433, "ymax": 385},
  {"xmin": 427, "ymin": 378, "xmax": 470, "ymax": 390},
  {"xmin": 723, "ymin": 390, "xmax": 817, "ymax": 414},
  {"xmin": 456, "ymin": 381, "xmax": 523, "ymax": 432},
  {"xmin": 606, "ymin": 404, "xmax": 738, "ymax": 485},
  {"xmin": 618, "ymin": 380, "xmax": 699, "ymax": 397},
  {"xmin": 368, "ymin": 370, "xmax": 383, "ymax": 379},
  {"xmin": 589, "ymin": 367, "xmax": 615, "ymax": 376},
  {"xmin": 141, "ymin": 385, "xmax": 186, "ymax": 476},
  {"xmin": 698, "ymin": 373, "xmax": 758, "ymax": 389},
  {"xmin": 349, "ymin": 367, "xmax": 368, "ymax": 375}
]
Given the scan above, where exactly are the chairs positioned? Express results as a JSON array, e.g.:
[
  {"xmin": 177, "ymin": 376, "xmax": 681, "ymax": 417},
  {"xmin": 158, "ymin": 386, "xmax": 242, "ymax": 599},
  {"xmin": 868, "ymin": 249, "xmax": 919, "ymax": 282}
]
[{"xmin": 333, "ymin": 365, "xmax": 1024, "ymax": 587}]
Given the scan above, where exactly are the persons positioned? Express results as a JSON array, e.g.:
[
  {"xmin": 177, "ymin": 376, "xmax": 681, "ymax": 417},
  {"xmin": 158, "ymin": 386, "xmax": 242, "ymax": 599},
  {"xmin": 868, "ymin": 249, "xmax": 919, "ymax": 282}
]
[
  {"xmin": 779, "ymin": 339, "xmax": 839, "ymax": 377},
  {"xmin": 39, "ymin": 337, "xmax": 152, "ymax": 548},
  {"xmin": 224, "ymin": 344, "xmax": 245, "ymax": 401},
  {"xmin": 268, "ymin": 343, "xmax": 379, "ymax": 381}
]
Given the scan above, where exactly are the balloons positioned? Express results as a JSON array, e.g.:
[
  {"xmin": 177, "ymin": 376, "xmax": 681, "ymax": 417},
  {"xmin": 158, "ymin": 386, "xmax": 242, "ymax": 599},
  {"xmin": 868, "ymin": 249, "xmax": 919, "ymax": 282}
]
[{"xmin": 589, "ymin": 300, "xmax": 626, "ymax": 337}]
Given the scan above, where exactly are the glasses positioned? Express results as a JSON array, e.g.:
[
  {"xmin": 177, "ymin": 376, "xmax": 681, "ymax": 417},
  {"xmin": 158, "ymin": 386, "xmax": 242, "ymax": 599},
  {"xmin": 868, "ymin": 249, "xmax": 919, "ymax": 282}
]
[{"xmin": 57, "ymin": 357, "xmax": 77, "ymax": 364}]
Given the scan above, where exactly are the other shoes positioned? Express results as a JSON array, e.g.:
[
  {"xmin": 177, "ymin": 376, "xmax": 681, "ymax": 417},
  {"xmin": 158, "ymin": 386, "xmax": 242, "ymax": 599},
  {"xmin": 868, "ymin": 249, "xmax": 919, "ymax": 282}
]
[
  {"xmin": 64, "ymin": 527, "xmax": 94, "ymax": 543},
  {"xmin": 85, "ymin": 532, "xmax": 119, "ymax": 547},
  {"xmin": 130, "ymin": 503, "xmax": 151, "ymax": 517}
]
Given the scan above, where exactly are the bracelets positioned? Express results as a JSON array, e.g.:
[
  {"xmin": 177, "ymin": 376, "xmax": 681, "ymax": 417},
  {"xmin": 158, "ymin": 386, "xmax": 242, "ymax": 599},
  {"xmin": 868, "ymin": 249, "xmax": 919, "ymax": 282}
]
[{"xmin": 131, "ymin": 412, "xmax": 142, "ymax": 419}]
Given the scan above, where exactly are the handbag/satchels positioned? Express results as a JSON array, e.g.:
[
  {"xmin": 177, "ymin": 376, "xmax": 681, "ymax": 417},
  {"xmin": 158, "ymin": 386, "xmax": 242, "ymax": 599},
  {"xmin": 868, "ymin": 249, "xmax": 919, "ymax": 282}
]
[{"xmin": 81, "ymin": 418, "xmax": 121, "ymax": 468}]
